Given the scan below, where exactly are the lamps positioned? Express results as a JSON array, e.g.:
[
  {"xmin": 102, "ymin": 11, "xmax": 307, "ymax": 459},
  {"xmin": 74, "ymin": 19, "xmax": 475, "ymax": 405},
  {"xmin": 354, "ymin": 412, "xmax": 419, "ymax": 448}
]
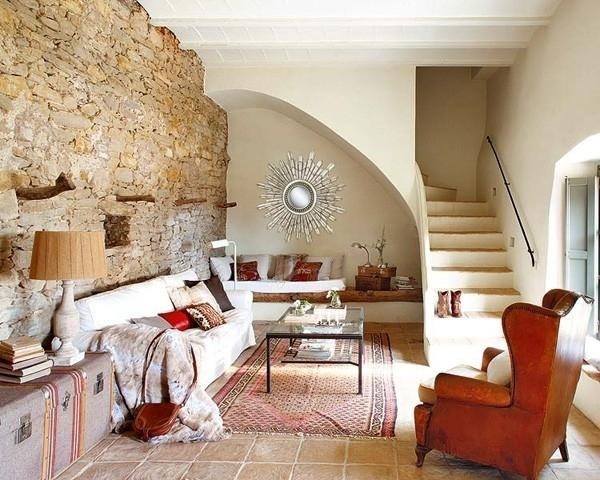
[
  {"xmin": 352, "ymin": 242, "xmax": 372, "ymax": 268},
  {"xmin": 28, "ymin": 231, "xmax": 107, "ymax": 367},
  {"xmin": 211, "ymin": 239, "xmax": 237, "ymax": 282}
]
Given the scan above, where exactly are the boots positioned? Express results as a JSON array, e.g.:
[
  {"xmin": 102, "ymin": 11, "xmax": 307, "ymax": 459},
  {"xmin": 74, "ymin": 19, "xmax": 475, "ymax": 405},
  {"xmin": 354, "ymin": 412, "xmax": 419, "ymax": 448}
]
[
  {"xmin": 437, "ymin": 291, "xmax": 449, "ymax": 318},
  {"xmin": 451, "ymin": 290, "xmax": 461, "ymax": 316}
]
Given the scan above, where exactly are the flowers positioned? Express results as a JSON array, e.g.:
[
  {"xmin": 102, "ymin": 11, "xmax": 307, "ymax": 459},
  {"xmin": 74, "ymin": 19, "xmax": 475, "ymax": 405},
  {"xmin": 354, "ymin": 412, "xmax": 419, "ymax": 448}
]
[{"xmin": 375, "ymin": 224, "xmax": 388, "ymax": 259}]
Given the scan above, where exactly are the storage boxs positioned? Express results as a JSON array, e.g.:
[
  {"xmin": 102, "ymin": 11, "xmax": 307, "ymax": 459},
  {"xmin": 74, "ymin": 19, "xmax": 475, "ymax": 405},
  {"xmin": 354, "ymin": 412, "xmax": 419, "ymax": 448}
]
[
  {"xmin": 358, "ymin": 266, "xmax": 397, "ymax": 277},
  {"xmin": 355, "ymin": 275, "xmax": 390, "ymax": 291},
  {"xmin": 0, "ymin": 353, "xmax": 114, "ymax": 480}
]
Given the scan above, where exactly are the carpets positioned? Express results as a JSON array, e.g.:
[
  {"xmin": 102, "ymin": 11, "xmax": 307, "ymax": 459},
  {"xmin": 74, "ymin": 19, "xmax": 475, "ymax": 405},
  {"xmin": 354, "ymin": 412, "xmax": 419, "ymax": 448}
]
[{"xmin": 211, "ymin": 332, "xmax": 398, "ymax": 440}]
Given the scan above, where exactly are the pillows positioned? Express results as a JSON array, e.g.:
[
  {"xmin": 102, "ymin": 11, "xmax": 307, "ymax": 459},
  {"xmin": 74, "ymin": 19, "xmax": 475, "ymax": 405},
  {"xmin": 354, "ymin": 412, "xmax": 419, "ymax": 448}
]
[
  {"xmin": 486, "ymin": 351, "xmax": 512, "ymax": 386},
  {"xmin": 210, "ymin": 254, "xmax": 332, "ymax": 280},
  {"xmin": 130, "ymin": 274, "xmax": 235, "ymax": 333}
]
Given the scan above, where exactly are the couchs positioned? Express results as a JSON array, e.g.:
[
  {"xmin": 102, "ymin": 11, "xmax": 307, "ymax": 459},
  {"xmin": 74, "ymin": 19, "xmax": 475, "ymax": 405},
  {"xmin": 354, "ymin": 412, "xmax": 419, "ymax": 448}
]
[
  {"xmin": 76, "ymin": 269, "xmax": 253, "ymax": 413},
  {"xmin": 209, "ymin": 254, "xmax": 346, "ymax": 294}
]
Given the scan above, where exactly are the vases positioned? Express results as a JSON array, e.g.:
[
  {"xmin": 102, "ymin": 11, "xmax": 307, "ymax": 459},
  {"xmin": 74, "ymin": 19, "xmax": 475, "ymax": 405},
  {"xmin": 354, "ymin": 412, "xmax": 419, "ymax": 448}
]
[{"xmin": 379, "ymin": 252, "xmax": 383, "ymax": 268}]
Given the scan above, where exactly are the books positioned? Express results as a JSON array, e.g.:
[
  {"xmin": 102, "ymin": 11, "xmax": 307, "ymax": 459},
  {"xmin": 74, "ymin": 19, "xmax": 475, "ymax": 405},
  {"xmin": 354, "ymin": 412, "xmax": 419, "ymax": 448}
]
[
  {"xmin": 298, "ymin": 326, "xmax": 341, "ymax": 359},
  {"xmin": 284, "ymin": 314, "xmax": 322, "ymax": 324},
  {"xmin": 0, "ymin": 336, "xmax": 54, "ymax": 385},
  {"xmin": 391, "ymin": 275, "xmax": 417, "ymax": 291}
]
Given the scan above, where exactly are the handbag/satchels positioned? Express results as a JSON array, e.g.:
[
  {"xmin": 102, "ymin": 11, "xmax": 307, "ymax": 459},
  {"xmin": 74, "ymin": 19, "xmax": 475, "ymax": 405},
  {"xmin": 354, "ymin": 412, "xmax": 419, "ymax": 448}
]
[{"xmin": 132, "ymin": 403, "xmax": 182, "ymax": 443}]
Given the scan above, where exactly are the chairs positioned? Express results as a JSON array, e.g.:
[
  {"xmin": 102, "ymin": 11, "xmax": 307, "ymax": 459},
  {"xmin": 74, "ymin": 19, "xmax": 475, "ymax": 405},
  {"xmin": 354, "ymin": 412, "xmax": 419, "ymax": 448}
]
[{"xmin": 415, "ymin": 288, "xmax": 593, "ymax": 480}]
